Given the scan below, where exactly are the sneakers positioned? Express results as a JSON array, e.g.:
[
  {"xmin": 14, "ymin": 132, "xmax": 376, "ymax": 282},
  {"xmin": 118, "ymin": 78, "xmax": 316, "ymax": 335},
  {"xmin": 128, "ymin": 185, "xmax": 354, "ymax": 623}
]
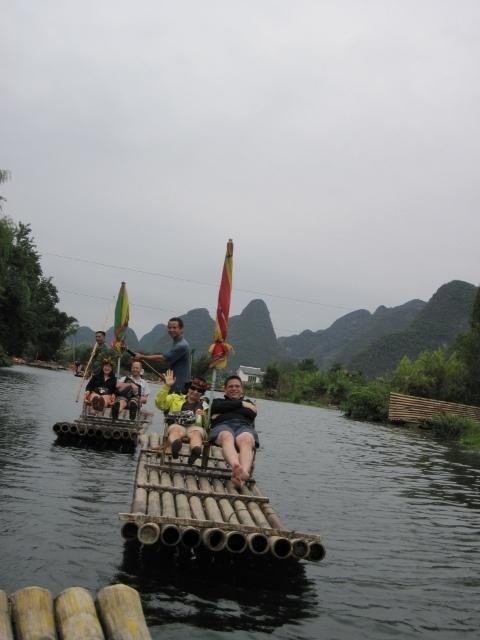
[
  {"xmin": 129, "ymin": 401, "xmax": 137, "ymax": 420},
  {"xmin": 172, "ymin": 439, "xmax": 181, "ymax": 459},
  {"xmin": 91, "ymin": 395, "xmax": 106, "ymax": 411},
  {"xmin": 188, "ymin": 447, "xmax": 201, "ymax": 464},
  {"xmin": 112, "ymin": 402, "xmax": 120, "ymax": 418}
]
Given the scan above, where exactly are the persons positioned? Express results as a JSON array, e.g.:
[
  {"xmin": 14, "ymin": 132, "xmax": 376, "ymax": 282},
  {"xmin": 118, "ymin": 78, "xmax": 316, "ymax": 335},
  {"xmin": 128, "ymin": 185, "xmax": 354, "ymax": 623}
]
[
  {"xmin": 85, "ymin": 360, "xmax": 116, "ymax": 413},
  {"xmin": 154, "ymin": 368, "xmax": 209, "ymax": 467},
  {"xmin": 210, "ymin": 376, "xmax": 260, "ymax": 486},
  {"xmin": 91, "ymin": 330, "xmax": 109, "ymax": 372},
  {"xmin": 134, "ymin": 317, "xmax": 193, "ymax": 396},
  {"xmin": 111, "ymin": 360, "xmax": 150, "ymax": 421}
]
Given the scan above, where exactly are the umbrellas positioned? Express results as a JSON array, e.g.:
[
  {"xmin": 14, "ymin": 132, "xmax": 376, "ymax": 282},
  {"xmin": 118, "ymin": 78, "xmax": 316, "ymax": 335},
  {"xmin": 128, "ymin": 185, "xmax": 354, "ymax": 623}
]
[
  {"xmin": 114, "ymin": 280, "xmax": 132, "ymax": 385},
  {"xmin": 204, "ymin": 238, "xmax": 235, "ymax": 409}
]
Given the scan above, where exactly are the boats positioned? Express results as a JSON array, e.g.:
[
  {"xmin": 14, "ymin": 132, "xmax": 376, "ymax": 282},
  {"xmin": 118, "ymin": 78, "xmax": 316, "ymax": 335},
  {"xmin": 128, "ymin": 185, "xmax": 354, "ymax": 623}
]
[
  {"xmin": 53, "ymin": 406, "xmax": 154, "ymax": 443},
  {"xmin": 121, "ymin": 539, "xmax": 319, "ymax": 635},
  {"xmin": 74, "ymin": 361, "xmax": 91, "ymax": 378},
  {"xmin": 52, "ymin": 436, "xmax": 138, "ymax": 458},
  {"xmin": 118, "ymin": 432, "xmax": 325, "ymax": 564},
  {"xmin": 0, "ymin": 583, "xmax": 155, "ymax": 640}
]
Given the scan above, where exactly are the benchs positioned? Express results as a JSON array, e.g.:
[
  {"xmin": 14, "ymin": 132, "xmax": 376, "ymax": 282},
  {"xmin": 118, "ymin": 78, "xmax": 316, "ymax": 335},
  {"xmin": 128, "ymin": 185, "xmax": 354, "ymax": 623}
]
[
  {"xmin": 82, "ymin": 391, "xmax": 142, "ymax": 426},
  {"xmin": 160, "ymin": 397, "xmax": 257, "ymax": 478}
]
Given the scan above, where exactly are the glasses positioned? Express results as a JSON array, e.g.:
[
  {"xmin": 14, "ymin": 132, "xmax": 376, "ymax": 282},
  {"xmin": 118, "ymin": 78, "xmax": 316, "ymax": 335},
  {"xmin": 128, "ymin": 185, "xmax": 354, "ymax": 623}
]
[{"xmin": 190, "ymin": 385, "xmax": 202, "ymax": 393}]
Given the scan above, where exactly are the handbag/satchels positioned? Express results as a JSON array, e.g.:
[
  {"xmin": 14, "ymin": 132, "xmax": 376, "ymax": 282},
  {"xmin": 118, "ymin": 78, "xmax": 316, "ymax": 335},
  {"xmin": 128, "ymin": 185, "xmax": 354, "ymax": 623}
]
[{"xmin": 165, "ymin": 410, "xmax": 195, "ymax": 424}]
